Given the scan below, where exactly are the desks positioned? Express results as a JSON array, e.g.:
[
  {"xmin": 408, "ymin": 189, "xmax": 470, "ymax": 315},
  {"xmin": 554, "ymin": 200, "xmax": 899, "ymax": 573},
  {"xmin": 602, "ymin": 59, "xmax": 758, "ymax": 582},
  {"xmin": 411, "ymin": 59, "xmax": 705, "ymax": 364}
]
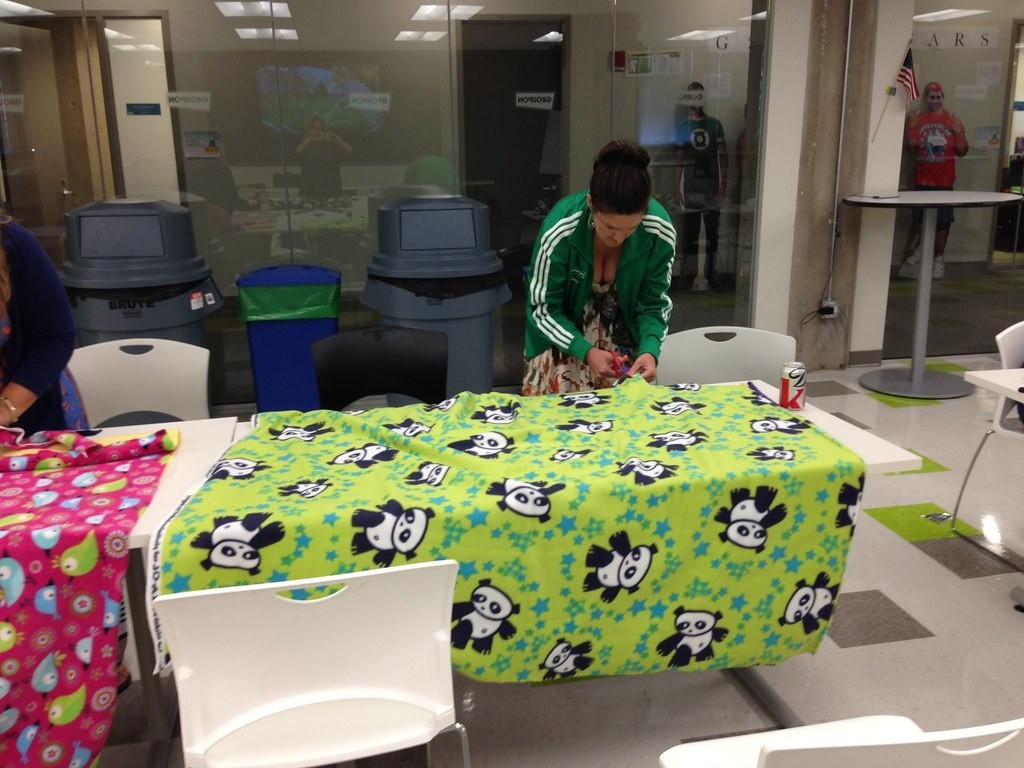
[
  {"xmin": 0, "ymin": 415, "xmax": 239, "ymax": 767},
  {"xmin": 842, "ymin": 193, "xmax": 1020, "ymax": 398},
  {"xmin": 226, "ymin": 380, "xmax": 923, "ymax": 667},
  {"xmin": 964, "ymin": 369, "xmax": 1024, "ymax": 404}
]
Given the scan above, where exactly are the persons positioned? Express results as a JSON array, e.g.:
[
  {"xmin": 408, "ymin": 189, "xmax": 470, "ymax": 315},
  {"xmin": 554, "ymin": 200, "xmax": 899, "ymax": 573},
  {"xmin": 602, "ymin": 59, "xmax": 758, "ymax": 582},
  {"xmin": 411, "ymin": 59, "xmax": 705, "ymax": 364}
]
[
  {"xmin": 0, "ymin": 214, "xmax": 77, "ymax": 440},
  {"xmin": 522, "ymin": 139, "xmax": 677, "ymax": 395},
  {"xmin": 906, "ymin": 82, "xmax": 969, "ymax": 280},
  {"xmin": 674, "ymin": 83, "xmax": 727, "ymax": 290},
  {"xmin": 295, "ymin": 117, "xmax": 351, "ymax": 199}
]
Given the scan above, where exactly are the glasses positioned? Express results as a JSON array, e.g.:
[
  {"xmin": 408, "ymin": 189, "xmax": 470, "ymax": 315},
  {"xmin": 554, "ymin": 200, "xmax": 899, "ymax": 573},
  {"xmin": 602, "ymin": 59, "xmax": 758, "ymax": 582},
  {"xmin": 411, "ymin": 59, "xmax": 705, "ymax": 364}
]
[{"xmin": 928, "ymin": 95, "xmax": 942, "ymax": 99}]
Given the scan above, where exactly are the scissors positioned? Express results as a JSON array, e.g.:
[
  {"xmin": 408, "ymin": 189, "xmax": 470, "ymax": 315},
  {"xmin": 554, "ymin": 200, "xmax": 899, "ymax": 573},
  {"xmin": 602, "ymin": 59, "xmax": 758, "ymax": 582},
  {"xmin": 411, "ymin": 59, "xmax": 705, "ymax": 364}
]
[{"xmin": 607, "ymin": 349, "xmax": 627, "ymax": 379}]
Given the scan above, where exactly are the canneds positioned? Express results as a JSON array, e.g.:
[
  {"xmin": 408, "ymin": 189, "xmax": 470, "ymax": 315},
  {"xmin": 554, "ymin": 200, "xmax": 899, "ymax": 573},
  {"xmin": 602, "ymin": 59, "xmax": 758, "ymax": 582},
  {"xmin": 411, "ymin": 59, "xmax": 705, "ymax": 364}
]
[{"xmin": 779, "ymin": 362, "xmax": 807, "ymax": 411}]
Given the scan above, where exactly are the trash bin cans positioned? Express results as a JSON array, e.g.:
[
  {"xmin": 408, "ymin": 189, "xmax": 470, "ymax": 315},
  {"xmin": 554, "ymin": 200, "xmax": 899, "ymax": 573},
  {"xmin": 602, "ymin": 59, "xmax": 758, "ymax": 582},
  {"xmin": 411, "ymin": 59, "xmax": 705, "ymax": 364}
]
[
  {"xmin": 236, "ymin": 263, "xmax": 343, "ymax": 414},
  {"xmin": 59, "ymin": 197, "xmax": 225, "ymax": 429},
  {"xmin": 359, "ymin": 195, "xmax": 512, "ymax": 403}
]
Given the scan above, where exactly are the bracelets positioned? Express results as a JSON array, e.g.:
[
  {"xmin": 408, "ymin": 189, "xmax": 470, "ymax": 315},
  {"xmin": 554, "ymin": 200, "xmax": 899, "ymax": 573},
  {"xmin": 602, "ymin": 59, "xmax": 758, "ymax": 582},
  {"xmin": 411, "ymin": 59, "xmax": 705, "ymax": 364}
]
[{"xmin": 0, "ymin": 395, "xmax": 18, "ymax": 423}]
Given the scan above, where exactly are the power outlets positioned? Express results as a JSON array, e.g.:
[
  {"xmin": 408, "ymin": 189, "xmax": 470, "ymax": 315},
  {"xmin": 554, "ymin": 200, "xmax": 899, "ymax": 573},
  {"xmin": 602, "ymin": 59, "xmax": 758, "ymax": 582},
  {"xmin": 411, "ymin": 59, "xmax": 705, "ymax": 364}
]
[{"xmin": 820, "ymin": 300, "xmax": 839, "ymax": 319}]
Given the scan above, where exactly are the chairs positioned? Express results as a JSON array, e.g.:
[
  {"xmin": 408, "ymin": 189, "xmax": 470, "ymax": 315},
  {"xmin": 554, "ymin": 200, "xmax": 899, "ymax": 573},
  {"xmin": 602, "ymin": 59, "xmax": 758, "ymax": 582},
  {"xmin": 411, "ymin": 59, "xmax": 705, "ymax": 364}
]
[
  {"xmin": 651, "ymin": 327, "xmax": 797, "ymax": 390},
  {"xmin": 309, "ymin": 324, "xmax": 447, "ymax": 411},
  {"xmin": 153, "ymin": 562, "xmax": 469, "ymax": 768},
  {"xmin": 659, "ymin": 714, "xmax": 1024, "ymax": 768},
  {"xmin": 952, "ymin": 320, "xmax": 1024, "ymax": 573},
  {"xmin": 65, "ymin": 338, "xmax": 210, "ymax": 428}
]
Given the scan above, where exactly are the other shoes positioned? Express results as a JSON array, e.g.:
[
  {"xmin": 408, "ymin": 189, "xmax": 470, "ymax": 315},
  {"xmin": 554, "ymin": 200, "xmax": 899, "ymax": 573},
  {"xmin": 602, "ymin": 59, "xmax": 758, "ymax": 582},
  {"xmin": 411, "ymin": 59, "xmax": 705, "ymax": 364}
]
[
  {"xmin": 906, "ymin": 251, "xmax": 922, "ymax": 265},
  {"xmin": 704, "ymin": 273, "xmax": 721, "ymax": 288},
  {"xmin": 933, "ymin": 256, "xmax": 945, "ymax": 278}
]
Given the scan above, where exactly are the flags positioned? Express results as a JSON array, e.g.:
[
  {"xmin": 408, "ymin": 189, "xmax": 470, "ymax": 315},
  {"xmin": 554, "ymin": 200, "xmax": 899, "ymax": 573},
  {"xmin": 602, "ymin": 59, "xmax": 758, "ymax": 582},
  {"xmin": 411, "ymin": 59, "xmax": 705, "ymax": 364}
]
[{"xmin": 897, "ymin": 48, "xmax": 921, "ymax": 104}]
